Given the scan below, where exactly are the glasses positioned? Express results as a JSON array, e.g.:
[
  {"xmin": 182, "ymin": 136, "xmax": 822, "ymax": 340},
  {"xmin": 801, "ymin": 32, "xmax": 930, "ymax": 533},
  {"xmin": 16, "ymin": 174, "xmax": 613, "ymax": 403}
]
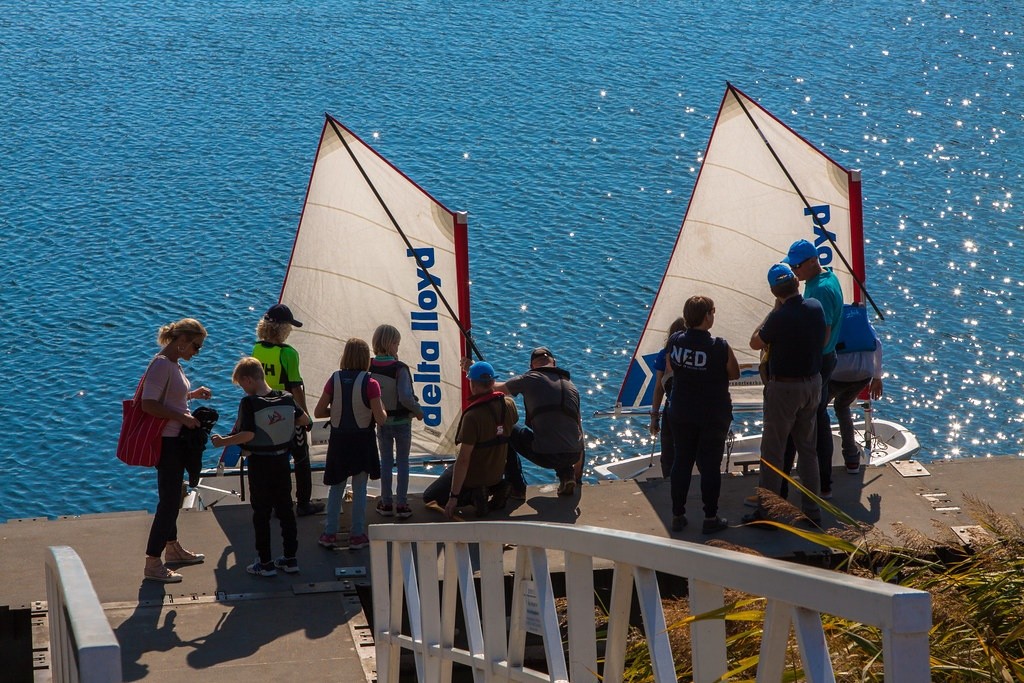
[
  {"xmin": 192, "ymin": 342, "xmax": 204, "ymax": 350},
  {"xmin": 703, "ymin": 306, "xmax": 717, "ymax": 314},
  {"xmin": 790, "ymin": 257, "xmax": 811, "ymax": 269}
]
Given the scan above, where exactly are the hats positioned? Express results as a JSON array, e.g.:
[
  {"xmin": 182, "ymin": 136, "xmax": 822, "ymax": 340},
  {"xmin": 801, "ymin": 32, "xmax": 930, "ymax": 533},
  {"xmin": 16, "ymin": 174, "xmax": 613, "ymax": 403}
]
[
  {"xmin": 264, "ymin": 304, "xmax": 302, "ymax": 327},
  {"xmin": 466, "ymin": 361, "xmax": 494, "ymax": 381},
  {"xmin": 784, "ymin": 241, "xmax": 817, "ymax": 266},
  {"xmin": 531, "ymin": 346, "xmax": 555, "ymax": 362},
  {"xmin": 766, "ymin": 261, "xmax": 793, "ymax": 286}
]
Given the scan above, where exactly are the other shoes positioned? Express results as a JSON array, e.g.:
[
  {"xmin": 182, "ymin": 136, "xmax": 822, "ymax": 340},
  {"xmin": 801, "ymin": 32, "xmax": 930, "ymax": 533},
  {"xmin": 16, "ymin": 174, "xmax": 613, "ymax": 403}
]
[
  {"xmin": 467, "ymin": 479, "xmax": 512, "ymax": 518},
  {"xmin": 297, "ymin": 502, "xmax": 325, "ymax": 516},
  {"xmin": 509, "ymin": 481, "xmax": 576, "ymax": 505}
]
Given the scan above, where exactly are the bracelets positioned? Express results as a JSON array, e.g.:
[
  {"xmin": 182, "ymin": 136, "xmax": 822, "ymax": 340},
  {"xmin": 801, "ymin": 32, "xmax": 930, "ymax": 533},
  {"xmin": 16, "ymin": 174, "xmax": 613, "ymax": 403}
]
[{"xmin": 650, "ymin": 409, "xmax": 660, "ymax": 418}]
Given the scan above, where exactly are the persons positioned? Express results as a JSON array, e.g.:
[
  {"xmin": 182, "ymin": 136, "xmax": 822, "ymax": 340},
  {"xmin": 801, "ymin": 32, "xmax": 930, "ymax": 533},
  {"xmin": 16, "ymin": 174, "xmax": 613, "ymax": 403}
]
[
  {"xmin": 649, "ymin": 317, "xmax": 687, "ymax": 479},
  {"xmin": 460, "ymin": 347, "xmax": 586, "ymax": 497},
  {"xmin": 366, "ymin": 324, "xmax": 424, "ymax": 517},
  {"xmin": 252, "ymin": 303, "xmax": 326, "ymax": 516},
  {"xmin": 212, "ymin": 357, "xmax": 312, "ymax": 576},
  {"xmin": 742, "ymin": 239, "xmax": 843, "ymax": 528},
  {"xmin": 142, "ymin": 317, "xmax": 212, "ymax": 583},
  {"xmin": 667, "ymin": 296, "xmax": 741, "ymax": 534},
  {"xmin": 827, "ymin": 302, "xmax": 883, "ymax": 474},
  {"xmin": 422, "ymin": 361, "xmax": 519, "ymax": 519},
  {"xmin": 314, "ymin": 337, "xmax": 387, "ymax": 550}
]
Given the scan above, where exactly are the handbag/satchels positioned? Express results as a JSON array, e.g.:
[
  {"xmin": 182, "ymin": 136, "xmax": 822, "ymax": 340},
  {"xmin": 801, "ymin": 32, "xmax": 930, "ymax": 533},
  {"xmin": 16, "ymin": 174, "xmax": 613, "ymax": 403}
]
[
  {"xmin": 670, "ymin": 394, "xmax": 689, "ymax": 426},
  {"xmin": 116, "ymin": 355, "xmax": 172, "ymax": 467}
]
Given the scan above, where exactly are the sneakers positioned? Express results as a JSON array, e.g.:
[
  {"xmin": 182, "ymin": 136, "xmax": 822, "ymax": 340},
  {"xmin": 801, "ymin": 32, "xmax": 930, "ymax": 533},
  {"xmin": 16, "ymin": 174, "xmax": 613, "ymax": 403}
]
[
  {"xmin": 165, "ymin": 542, "xmax": 205, "ymax": 563},
  {"xmin": 376, "ymin": 500, "xmax": 393, "ymax": 516},
  {"xmin": 348, "ymin": 535, "xmax": 369, "ymax": 548},
  {"xmin": 318, "ymin": 533, "xmax": 337, "ymax": 547},
  {"xmin": 274, "ymin": 556, "xmax": 299, "ymax": 572},
  {"xmin": 145, "ymin": 557, "xmax": 183, "ymax": 581},
  {"xmin": 247, "ymin": 558, "xmax": 276, "ymax": 575},
  {"xmin": 845, "ymin": 452, "xmax": 860, "ymax": 473},
  {"xmin": 671, "ymin": 480, "xmax": 834, "ymax": 534},
  {"xmin": 396, "ymin": 504, "xmax": 412, "ymax": 517}
]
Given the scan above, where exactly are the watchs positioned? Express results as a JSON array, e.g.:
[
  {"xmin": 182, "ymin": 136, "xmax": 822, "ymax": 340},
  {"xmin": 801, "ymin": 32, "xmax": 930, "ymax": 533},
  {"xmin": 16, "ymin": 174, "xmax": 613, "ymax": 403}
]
[{"xmin": 449, "ymin": 492, "xmax": 459, "ymax": 498}]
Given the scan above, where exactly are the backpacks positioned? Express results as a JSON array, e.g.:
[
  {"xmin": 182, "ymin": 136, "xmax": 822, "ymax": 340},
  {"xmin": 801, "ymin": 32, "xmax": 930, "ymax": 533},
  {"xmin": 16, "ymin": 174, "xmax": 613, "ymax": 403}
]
[{"xmin": 835, "ymin": 302, "xmax": 876, "ymax": 353}]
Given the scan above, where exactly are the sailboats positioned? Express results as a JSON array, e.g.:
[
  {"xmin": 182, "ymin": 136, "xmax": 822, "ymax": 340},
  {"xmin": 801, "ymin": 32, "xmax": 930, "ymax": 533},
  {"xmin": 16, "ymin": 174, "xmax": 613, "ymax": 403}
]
[
  {"xmin": 591, "ymin": 79, "xmax": 922, "ymax": 482},
  {"xmin": 179, "ymin": 112, "xmax": 479, "ymax": 510}
]
